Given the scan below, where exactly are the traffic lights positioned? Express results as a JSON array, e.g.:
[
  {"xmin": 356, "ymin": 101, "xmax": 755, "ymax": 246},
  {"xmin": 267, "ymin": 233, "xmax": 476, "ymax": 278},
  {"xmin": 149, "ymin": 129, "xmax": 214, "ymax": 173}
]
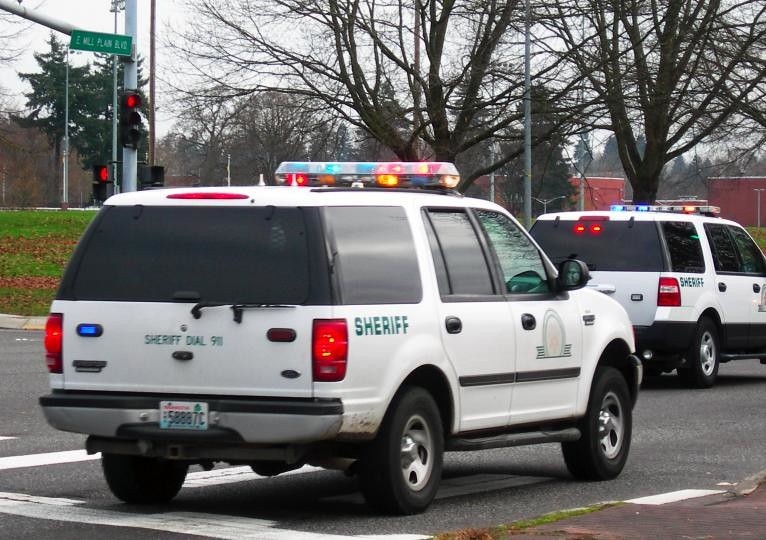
[
  {"xmin": 142, "ymin": 166, "xmax": 164, "ymax": 184},
  {"xmin": 119, "ymin": 91, "xmax": 142, "ymax": 148},
  {"xmin": 92, "ymin": 164, "xmax": 115, "ymax": 201}
]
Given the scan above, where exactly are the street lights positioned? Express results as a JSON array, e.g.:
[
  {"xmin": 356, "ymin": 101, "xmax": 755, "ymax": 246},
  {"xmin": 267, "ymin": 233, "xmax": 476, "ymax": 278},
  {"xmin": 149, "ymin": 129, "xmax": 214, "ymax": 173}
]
[
  {"xmin": 753, "ymin": 188, "xmax": 765, "ymax": 228},
  {"xmin": 108, "ymin": 0, "xmax": 130, "ymax": 195},
  {"xmin": 489, "ymin": 60, "xmax": 515, "ymax": 204},
  {"xmin": 532, "ymin": 195, "xmax": 566, "ymax": 214}
]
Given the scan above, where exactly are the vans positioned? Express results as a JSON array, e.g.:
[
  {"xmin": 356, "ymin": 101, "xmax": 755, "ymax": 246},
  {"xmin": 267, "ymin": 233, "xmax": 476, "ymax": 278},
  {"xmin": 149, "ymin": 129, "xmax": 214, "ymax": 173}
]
[
  {"xmin": 37, "ymin": 161, "xmax": 644, "ymax": 516},
  {"xmin": 526, "ymin": 199, "xmax": 766, "ymax": 386}
]
